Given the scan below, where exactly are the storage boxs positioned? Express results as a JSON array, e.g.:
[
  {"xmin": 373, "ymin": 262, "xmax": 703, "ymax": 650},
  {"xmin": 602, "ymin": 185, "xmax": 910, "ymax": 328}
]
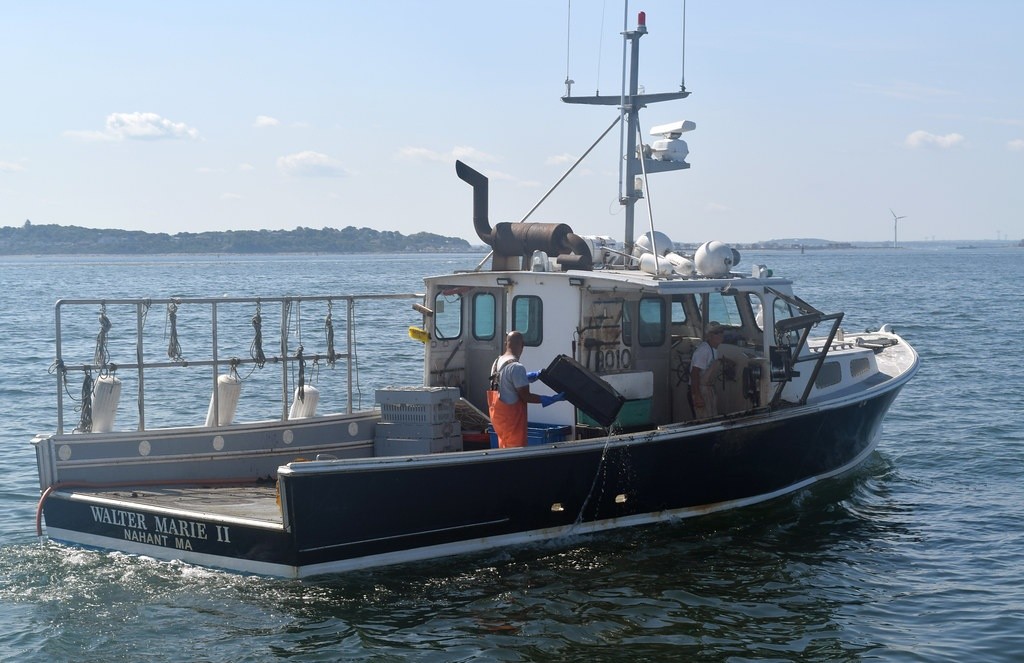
[
  {"xmin": 537, "ymin": 355, "xmax": 626, "ymax": 427},
  {"xmin": 374, "ymin": 386, "xmax": 463, "ymax": 455},
  {"xmin": 486, "ymin": 422, "xmax": 571, "ymax": 449}
]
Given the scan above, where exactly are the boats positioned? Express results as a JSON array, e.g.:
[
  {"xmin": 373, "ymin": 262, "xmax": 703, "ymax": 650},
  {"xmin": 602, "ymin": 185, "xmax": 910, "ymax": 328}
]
[{"xmin": 30, "ymin": 0, "xmax": 922, "ymax": 589}]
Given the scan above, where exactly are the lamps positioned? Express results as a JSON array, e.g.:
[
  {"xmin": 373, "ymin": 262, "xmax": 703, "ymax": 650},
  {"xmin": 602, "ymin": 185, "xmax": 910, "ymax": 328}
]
[
  {"xmin": 497, "ymin": 277, "xmax": 511, "ymax": 286},
  {"xmin": 569, "ymin": 278, "xmax": 583, "ymax": 287}
]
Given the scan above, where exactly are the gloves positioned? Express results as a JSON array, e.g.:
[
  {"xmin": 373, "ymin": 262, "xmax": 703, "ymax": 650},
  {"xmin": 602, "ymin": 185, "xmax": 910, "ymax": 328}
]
[
  {"xmin": 541, "ymin": 390, "xmax": 566, "ymax": 407},
  {"xmin": 526, "ymin": 368, "xmax": 546, "ymax": 383}
]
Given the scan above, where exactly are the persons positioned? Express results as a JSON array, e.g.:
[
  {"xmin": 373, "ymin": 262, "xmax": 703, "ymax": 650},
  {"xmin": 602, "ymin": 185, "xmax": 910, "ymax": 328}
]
[
  {"xmin": 487, "ymin": 331, "xmax": 566, "ymax": 448},
  {"xmin": 689, "ymin": 320, "xmax": 723, "ymax": 420}
]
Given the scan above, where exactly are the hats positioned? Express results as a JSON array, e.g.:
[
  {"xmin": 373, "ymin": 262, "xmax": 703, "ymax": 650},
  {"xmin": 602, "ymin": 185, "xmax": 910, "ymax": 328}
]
[{"xmin": 705, "ymin": 321, "xmax": 725, "ymax": 335}]
